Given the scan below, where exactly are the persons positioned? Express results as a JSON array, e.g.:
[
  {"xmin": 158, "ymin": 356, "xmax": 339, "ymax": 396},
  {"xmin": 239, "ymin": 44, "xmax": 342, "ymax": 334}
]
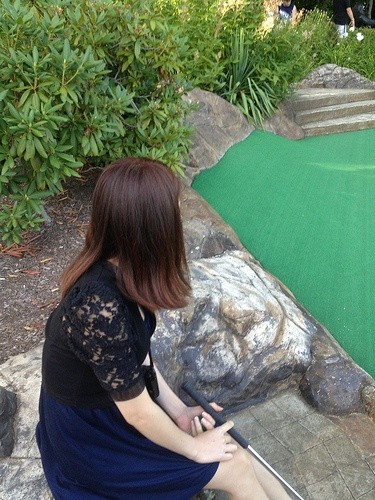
[
  {"xmin": 34, "ymin": 156, "xmax": 291, "ymax": 500},
  {"xmin": 279, "ymin": 0, "xmax": 355, "ymax": 39}
]
